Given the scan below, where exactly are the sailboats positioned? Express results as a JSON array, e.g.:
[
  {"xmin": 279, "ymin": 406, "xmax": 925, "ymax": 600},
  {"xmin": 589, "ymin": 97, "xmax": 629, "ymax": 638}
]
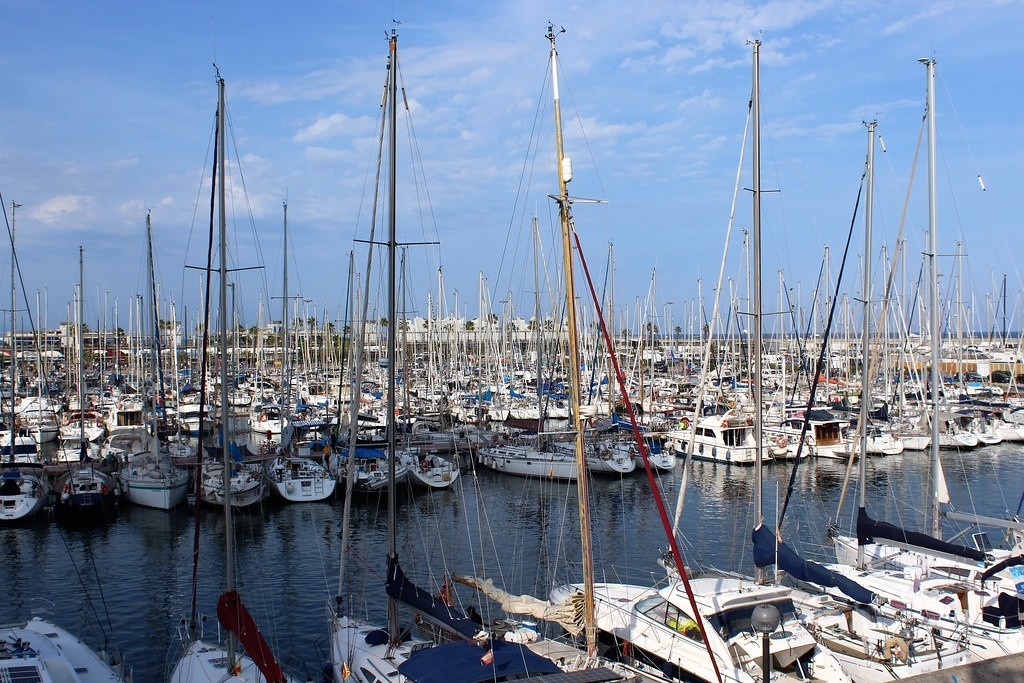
[{"xmin": 0, "ymin": 32, "xmax": 1024, "ymax": 681}]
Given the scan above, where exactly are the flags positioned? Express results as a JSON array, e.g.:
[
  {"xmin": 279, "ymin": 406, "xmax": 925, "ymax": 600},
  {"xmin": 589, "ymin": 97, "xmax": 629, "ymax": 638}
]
[
  {"xmin": 235, "ymin": 661, "xmax": 241, "ymax": 675},
  {"xmin": 342, "ymin": 662, "xmax": 350, "ymax": 680},
  {"xmin": 441, "ymin": 574, "xmax": 446, "ymax": 584},
  {"xmin": 482, "ymin": 649, "xmax": 494, "ymax": 665}
]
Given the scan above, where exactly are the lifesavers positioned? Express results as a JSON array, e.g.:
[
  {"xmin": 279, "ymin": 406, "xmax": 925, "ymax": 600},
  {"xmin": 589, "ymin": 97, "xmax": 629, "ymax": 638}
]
[
  {"xmin": 97, "ymin": 421, "xmax": 102, "ymax": 427},
  {"xmin": 260, "ymin": 413, "xmax": 267, "ymax": 422},
  {"xmin": 777, "ymin": 437, "xmax": 787, "ymax": 448},
  {"xmin": 884, "ymin": 638, "xmax": 907, "ymax": 661},
  {"xmin": 37, "ymin": 450, "xmax": 42, "ymax": 461},
  {"xmin": 247, "ymin": 475, "xmax": 257, "ymax": 482},
  {"xmin": 414, "ymin": 594, "xmax": 454, "ymax": 630},
  {"xmin": 102, "ymin": 485, "xmax": 108, "ymax": 495},
  {"xmin": 601, "ymin": 449, "xmax": 612, "ymax": 460},
  {"xmin": 420, "ymin": 460, "xmax": 431, "ymax": 472}
]
